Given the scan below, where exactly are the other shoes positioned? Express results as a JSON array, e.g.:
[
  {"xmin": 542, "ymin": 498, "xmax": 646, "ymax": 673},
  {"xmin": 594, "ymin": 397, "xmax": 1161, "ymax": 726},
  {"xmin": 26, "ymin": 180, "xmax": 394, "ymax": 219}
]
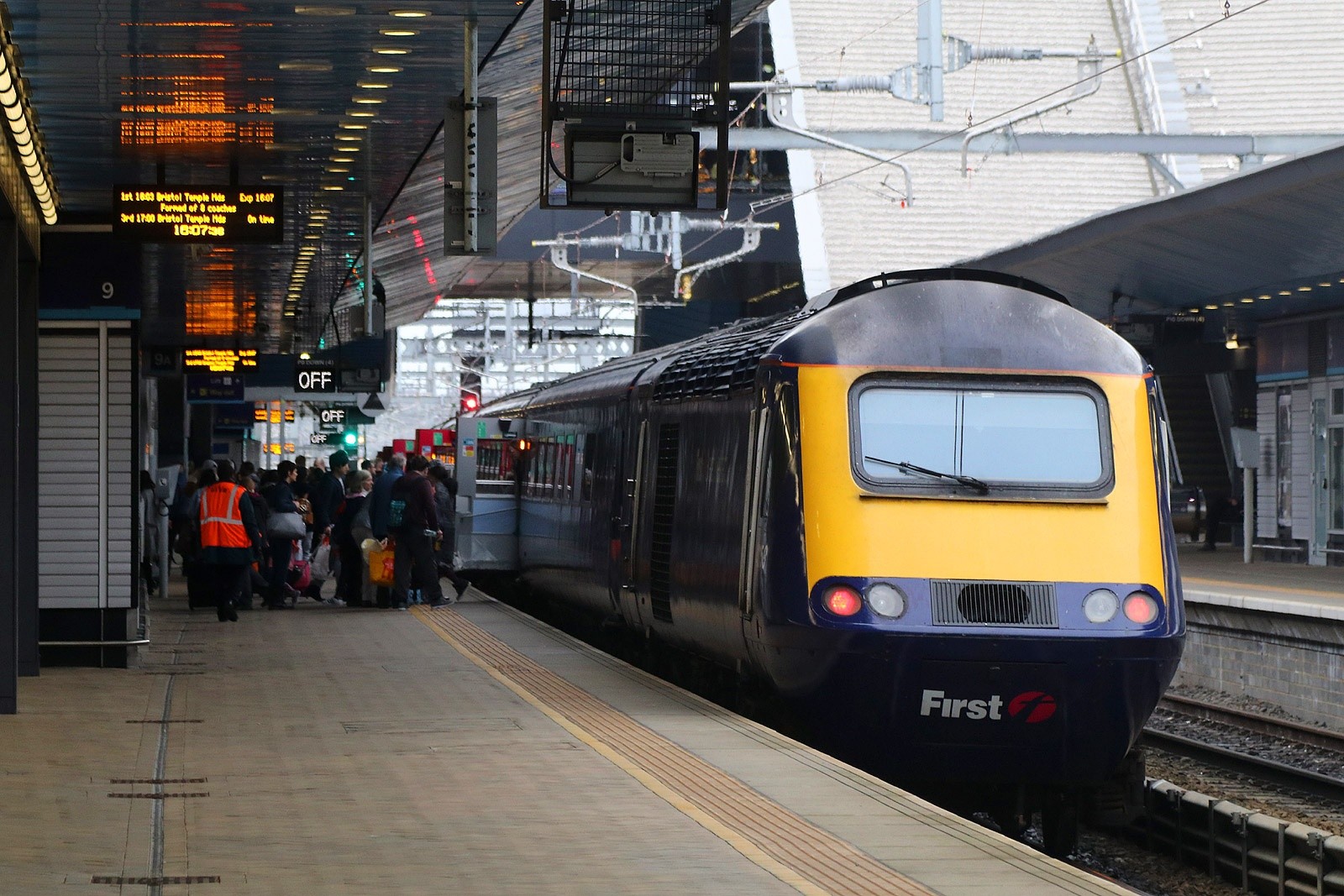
[
  {"xmin": 398, "ymin": 602, "xmax": 410, "ymax": 611},
  {"xmin": 261, "ymin": 596, "xmax": 286, "ymax": 609},
  {"xmin": 452, "ymin": 576, "xmax": 471, "ymax": 601},
  {"xmin": 430, "ymin": 597, "xmax": 455, "ymax": 609},
  {"xmin": 291, "ymin": 590, "xmax": 301, "ymax": 604},
  {"xmin": 331, "ymin": 596, "xmax": 391, "ymax": 609},
  {"xmin": 216, "ymin": 601, "xmax": 238, "ymax": 622},
  {"xmin": 305, "ymin": 583, "xmax": 327, "ymax": 601}
]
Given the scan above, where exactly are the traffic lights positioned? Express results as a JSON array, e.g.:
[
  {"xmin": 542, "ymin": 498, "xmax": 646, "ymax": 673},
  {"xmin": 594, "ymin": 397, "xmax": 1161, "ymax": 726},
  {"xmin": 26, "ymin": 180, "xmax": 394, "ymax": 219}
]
[
  {"xmin": 341, "ymin": 425, "xmax": 359, "ymax": 457},
  {"xmin": 459, "ymin": 372, "xmax": 481, "ymax": 414}
]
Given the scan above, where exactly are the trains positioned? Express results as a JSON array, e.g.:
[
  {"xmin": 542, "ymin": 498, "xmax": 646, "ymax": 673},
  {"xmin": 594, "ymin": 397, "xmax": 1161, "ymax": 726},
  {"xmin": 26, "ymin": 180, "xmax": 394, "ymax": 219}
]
[{"xmin": 375, "ymin": 267, "xmax": 1189, "ymax": 815}]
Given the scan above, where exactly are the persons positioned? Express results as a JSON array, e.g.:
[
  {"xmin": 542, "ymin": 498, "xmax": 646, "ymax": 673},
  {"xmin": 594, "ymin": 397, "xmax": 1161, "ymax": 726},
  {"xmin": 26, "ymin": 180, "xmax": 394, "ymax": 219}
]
[
  {"xmin": 165, "ymin": 446, "xmax": 472, "ymax": 610},
  {"xmin": 193, "ymin": 459, "xmax": 263, "ymax": 623},
  {"xmin": 606, "ymin": 523, "xmax": 625, "ymax": 617},
  {"xmin": 1202, "ymin": 464, "xmax": 1231, "ymax": 552}
]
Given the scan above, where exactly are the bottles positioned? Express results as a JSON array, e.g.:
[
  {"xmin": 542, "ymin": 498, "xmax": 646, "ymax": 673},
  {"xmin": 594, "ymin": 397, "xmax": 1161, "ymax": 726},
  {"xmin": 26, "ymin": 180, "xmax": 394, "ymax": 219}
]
[{"xmin": 423, "ymin": 529, "xmax": 439, "ymax": 538}]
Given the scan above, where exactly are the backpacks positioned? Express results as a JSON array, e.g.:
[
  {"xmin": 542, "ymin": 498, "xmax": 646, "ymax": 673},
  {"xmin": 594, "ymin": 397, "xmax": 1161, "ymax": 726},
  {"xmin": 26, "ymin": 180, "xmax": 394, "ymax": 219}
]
[{"xmin": 385, "ymin": 475, "xmax": 426, "ymax": 534}]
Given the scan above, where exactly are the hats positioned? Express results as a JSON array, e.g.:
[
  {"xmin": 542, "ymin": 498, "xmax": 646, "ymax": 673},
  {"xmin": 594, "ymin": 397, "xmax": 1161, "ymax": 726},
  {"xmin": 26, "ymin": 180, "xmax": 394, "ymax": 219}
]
[{"xmin": 201, "ymin": 459, "xmax": 220, "ymax": 471}]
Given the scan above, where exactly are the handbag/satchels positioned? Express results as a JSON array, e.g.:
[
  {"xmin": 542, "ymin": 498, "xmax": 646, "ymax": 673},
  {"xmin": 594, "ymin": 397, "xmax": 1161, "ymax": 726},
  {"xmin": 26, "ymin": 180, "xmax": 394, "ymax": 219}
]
[
  {"xmin": 367, "ymin": 545, "xmax": 396, "ymax": 588},
  {"xmin": 265, "ymin": 509, "xmax": 306, "ymax": 541},
  {"xmin": 308, "ymin": 536, "xmax": 329, "ymax": 581}
]
